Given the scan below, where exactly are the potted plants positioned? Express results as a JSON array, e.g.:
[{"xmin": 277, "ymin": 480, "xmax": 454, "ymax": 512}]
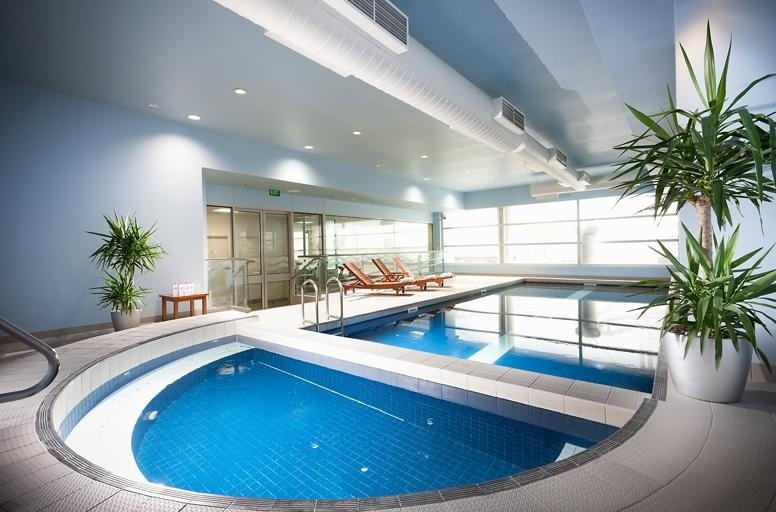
[
  {"xmin": 606, "ymin": 17, "xmax": 776, "ymax": 404},
  {"xmin": 86, "ymin": 208, "xmax": 171, "ymax": 332}
]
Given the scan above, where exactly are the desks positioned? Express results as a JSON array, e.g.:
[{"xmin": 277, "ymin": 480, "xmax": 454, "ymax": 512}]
[{"xmin": 159, "ymin": 292, "xmax": 210, "ymax": 321}]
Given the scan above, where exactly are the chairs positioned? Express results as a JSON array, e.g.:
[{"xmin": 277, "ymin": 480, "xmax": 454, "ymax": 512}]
[{"xmin": 341, "ymin": 257, "xmax": 454, "ymax": 296}]
